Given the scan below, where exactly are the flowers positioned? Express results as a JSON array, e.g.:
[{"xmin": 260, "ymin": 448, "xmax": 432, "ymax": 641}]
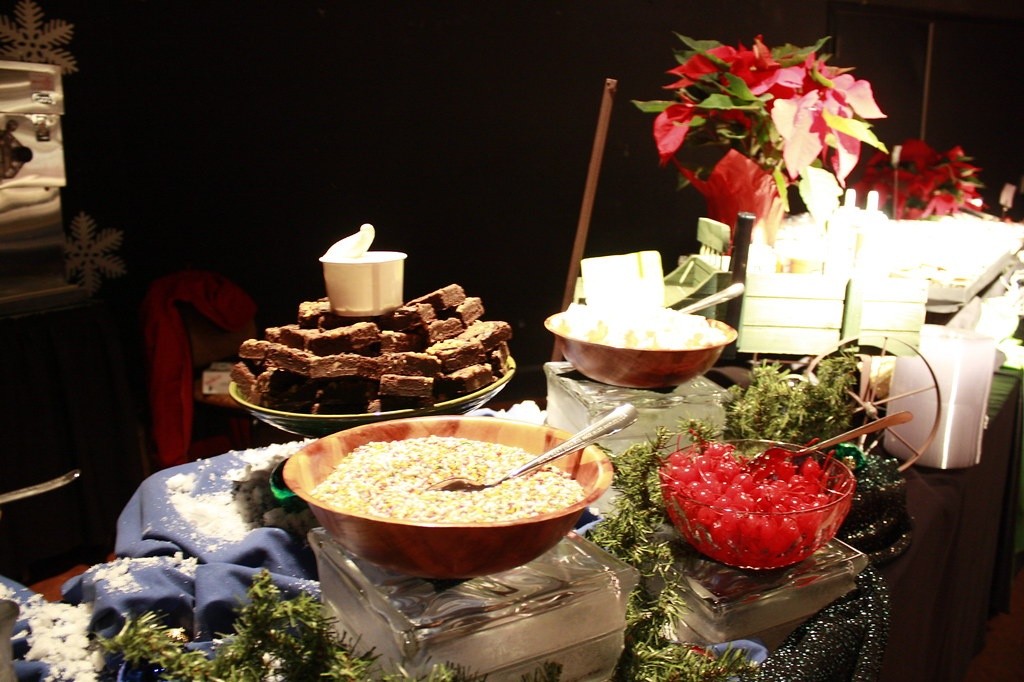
[
  {"xmin": 629, "ymin": 31, "xmax": 889, "ymax": 255},
  {"xmin": 851, "ymin": 140, "xmax": 990, "ymax": 220}
]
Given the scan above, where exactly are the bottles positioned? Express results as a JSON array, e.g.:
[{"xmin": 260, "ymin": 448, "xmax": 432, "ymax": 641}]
[{"xmin": 750, "ymin": 187, "xmax": 893, "ymax": 282}]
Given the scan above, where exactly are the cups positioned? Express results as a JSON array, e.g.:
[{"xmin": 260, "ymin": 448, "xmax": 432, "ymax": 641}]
[{"xmin": 883, "ymin": 324, "xmax": 995, "ymax": 469}]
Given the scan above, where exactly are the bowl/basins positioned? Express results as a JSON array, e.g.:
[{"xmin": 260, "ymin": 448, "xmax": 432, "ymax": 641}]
[
  {"xmin": 282, "ymin": 416, "xmax": 614, "ymax": 573},
  {"xmin": 229, "ymin": 354, "xmax": 517, "ymax": 436},
  {"xmin": 659, "ymin": 437, "xmax": 856, "ymax": 570},
  {"xmin": 543, "ymin": 309, "xmax": 739, "ymax": 390},
  {"xmin": 320, "ymin": 251, "xmax": 408, "ymax": 316}
]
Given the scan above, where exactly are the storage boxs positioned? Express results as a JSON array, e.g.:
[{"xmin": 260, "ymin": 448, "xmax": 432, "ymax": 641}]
[{"xmin": 693, "ymin": 215, "xmax": 930, "ymax": 357}]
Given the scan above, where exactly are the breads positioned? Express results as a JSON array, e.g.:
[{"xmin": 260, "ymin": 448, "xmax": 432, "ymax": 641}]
[{"xmin": 231, "ymin": 284, "xmax": 515, "ymax": 415}]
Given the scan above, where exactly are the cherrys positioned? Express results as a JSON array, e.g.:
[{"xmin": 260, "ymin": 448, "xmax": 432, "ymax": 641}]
[{"xmin": 649, "ymin": 430, "xmax": 852, "ymax": 565}]
